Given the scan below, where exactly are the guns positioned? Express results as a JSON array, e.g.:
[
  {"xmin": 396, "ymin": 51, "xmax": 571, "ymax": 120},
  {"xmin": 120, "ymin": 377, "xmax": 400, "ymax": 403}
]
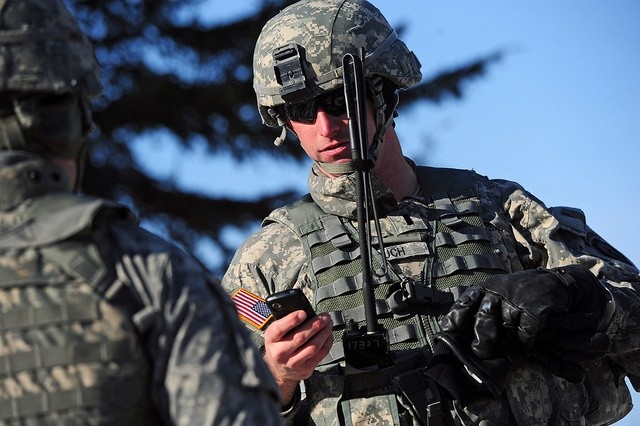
[{"xmin": 385, "ymin": 276, "xmax": 468, "ymax": 316}]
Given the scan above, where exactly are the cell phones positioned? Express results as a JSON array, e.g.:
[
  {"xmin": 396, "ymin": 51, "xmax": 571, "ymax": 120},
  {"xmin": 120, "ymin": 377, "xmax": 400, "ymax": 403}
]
[{"xmin": 263, "ymin": 287, "xmax": 318, "ymax": 336}]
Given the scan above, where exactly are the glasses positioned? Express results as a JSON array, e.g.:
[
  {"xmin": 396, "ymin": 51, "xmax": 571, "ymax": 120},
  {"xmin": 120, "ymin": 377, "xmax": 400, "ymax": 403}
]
[{"xmin": 283, "ymin": 88, "xmax": 354, "ymax": 126}]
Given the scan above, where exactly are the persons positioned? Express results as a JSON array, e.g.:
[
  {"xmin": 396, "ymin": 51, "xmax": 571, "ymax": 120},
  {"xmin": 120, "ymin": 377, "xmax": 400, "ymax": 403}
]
[
  {"xmin": 0, "ymin": 0, "xmax": 280, "ymax": 426},
  {"xmin": 220, "ymin": 0, "xmax": 640, "ymax": 426}
]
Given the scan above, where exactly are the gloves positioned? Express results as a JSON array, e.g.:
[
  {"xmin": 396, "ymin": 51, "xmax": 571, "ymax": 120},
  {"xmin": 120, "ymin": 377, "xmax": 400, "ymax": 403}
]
[
  {"xmin": 438, "ymin": 264, "xmax": 607, "ymax": 360},
  {"xmin": 423, "ymin": 317, "xmax": 610, "ymax": 411}
]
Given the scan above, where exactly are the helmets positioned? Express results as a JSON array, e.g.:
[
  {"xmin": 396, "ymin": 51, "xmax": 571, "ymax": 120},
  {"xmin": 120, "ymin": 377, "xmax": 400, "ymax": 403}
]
[
  {"xmin": 252, "ymin": 2, "xmax": 423, "ymax": 179},
  {"xmin": 1, "ymin": 0, "xmax": 104, "ymax": 192}
]
